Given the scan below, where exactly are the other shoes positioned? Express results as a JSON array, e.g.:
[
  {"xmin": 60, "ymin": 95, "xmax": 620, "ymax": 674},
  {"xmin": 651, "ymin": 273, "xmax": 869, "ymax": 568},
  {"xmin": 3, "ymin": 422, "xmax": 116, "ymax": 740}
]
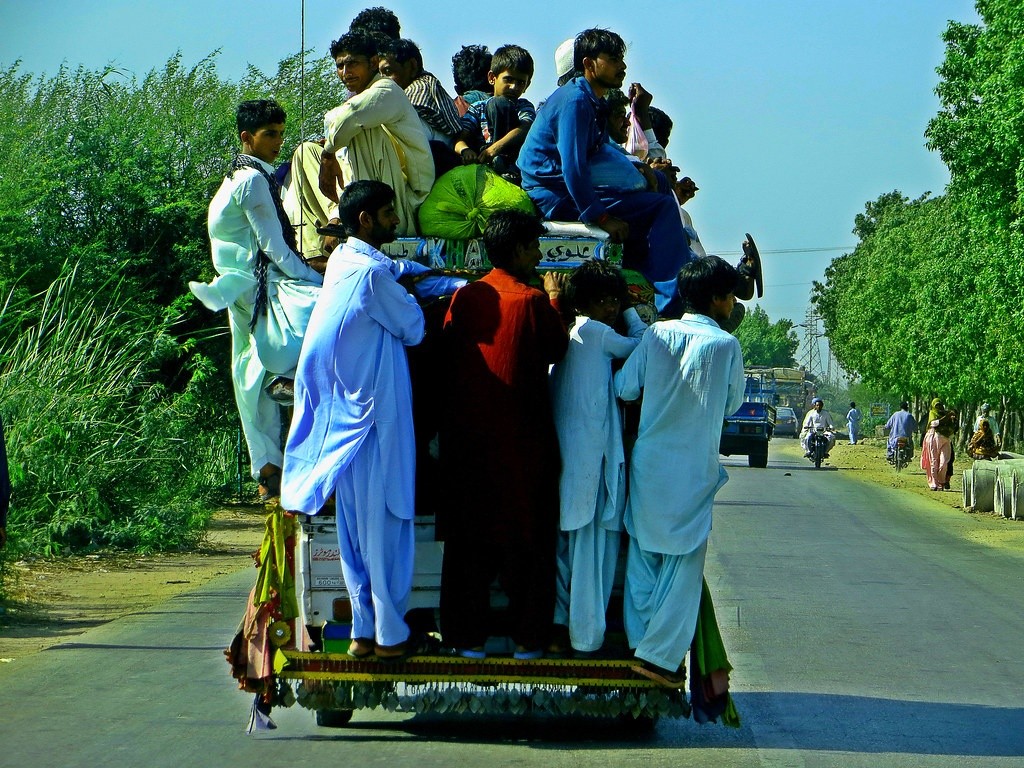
[
  {"xmin": 440, "ymin": 641, "xmax": 487, "ymax": 658},
  {"xmin": 316, "ymin": 222, "xmax": 347, "ymax": 237},
  {"xmin": 803, "ymin": 452, "xmax": 811, "ymax": 458},
  {"xmin": 346, "ymin": 637, "xmax": 375, "ymax": 659},
  {"xmin": 825, "ymin": 453, "xmax": 830, "ymax": 458},
  {"xmin": 514, "ymin": 645, "xmax": 545, "ymax": 660},
  {"xmin": 375, "ymin": 635, "xmax": 432, "ymax": 659},
  {"xmin": 945, "ymin": 480, "xmax": 951, "ymax": 489}
]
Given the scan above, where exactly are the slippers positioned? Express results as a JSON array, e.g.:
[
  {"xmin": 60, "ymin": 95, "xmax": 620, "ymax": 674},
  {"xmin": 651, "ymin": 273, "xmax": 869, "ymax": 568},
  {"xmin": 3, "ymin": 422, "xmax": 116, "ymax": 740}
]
[
  {"xmin": 741, "ymin": 232, "xmax": 765, "ymax": 298},
  {"xmin": 632, "ymin": 661, "xmax": 684, "ymax": 688}
]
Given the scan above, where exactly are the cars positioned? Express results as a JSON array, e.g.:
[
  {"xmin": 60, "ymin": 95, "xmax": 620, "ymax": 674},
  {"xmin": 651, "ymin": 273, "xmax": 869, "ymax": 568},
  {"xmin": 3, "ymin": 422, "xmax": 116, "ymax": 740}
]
[{"xmin": 772, "ymin": 406, "xmax": 799, "ymax": 438}]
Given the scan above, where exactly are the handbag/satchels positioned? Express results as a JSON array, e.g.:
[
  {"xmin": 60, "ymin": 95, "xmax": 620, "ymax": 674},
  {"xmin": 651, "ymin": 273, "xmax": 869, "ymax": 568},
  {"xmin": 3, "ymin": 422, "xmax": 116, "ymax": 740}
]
[
  {"xmin": 967, "ymin": 441, "xmax": 976, "ymax": 457},
  {"xmin": 622, "ymin": 105, "xmax": 649, "ymax": 160}
]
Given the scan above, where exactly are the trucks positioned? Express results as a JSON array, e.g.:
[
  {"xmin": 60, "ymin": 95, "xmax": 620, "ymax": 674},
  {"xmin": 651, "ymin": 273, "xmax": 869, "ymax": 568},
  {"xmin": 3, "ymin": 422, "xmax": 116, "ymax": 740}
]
[{"xmin": 716, "ymin": 370, "xmax": 780, "ymax": 467}]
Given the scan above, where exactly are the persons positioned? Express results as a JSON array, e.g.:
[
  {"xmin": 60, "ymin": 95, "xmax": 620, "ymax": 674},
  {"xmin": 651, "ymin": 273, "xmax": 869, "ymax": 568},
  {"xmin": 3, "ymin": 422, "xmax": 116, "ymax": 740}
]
[
  {"xmin": 884, "ymin": 401, "xmax": 918, "ymax": 462},
  {"xmin": 920, "ymin": 398, "xmax": 958, "ymax": 491},
  {"xmin": 276, "ymin": 178, "xmax": 467, "ymax": 660},
  {"xmin": 970, "ymin": 403, "xmax": 1001, "ymax": 460},
  {"xmin": 799, "ymin": 398, "xmax": 836, "ymax": 458},
  {"xmin": 188, "ymin": 101, "xmax": 324, "ymax": 503},
  {"xmin": 846, "ymin": 402, "xmax": 862, "ymax": 445},
  {"xmin": 613, "ymin": 255, "xmax": 746, "ymax": 676},
  {"xmin": 290, "ymin": 6, "xmax": 765, "ymax": 334},
  {"xmin": 547, "ymin": 255, "xmax": 650, "ymax": 658},
  {"xmin": 432, "ymin": 208, "xmax": 570, "ymax": 659}
]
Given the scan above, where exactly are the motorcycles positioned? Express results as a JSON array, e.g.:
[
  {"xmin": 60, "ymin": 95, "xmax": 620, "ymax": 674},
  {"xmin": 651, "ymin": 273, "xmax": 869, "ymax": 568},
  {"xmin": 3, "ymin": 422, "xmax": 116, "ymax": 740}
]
[
  {"xmin": 890, "ymin": 428, "xmax": 920, "ymax": 473},
  {"xmin": 804, "ymin": 426, "xmax": 831, "ymax": 468}
]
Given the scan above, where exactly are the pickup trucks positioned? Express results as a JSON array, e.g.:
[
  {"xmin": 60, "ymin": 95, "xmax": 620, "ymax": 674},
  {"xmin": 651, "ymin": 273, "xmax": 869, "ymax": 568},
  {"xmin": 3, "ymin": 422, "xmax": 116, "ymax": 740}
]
[{"xmin": 270, "ymin": 234, "xmax": 688, "ymax": 725}]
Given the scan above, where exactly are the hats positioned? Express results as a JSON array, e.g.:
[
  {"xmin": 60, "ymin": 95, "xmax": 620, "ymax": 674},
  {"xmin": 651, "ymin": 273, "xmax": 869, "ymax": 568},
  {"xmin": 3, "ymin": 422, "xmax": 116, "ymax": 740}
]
[
  {"xmin": 932, "ymin": 398, "xmax": 945, "ymax": 411},
  {"xmin": 812, "ymin": 397, "xmax": 822, "ymax": 404},
  {"xmin": 982, "ymin": 403, "xmax": 991, "ymax": 411},
  {"xmin": 555, "ymin": 38, "xmax": 574, "ymax": 87}
]
